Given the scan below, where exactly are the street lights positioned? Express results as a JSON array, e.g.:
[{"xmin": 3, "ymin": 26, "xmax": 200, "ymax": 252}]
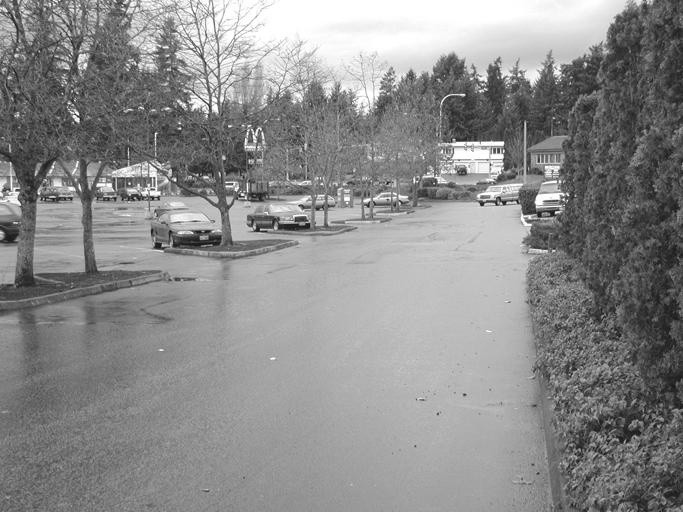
[{"xmin": 439, "ymin": 93, "xmax": 466, "ymax": 139}]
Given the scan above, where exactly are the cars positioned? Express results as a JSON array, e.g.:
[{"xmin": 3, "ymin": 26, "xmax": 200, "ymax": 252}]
[
  {"xmin": 476, "ymin": 183, "xmax": 523, "ymax": 206},
  {"xmin": 150, "ymin": 201, "xmax": 223, "ymax": 249},
  {"xmin": 535, "ymin": 180, "xmax": 571, "ymax": 217},
  {"xmin": 96, "ymin": 184, "xmax": 162, "ymax": 201},
  {"xmin": 247, "ymin": 194, "xmax": 335, "ymax": 232},
  {"xmin": 363, "ymin": 192, "xmax": 410, "ymax": 208},
  {"xmin": 299, "ymin": 181, "xmax": 312, "ymax": 186},
  {"xmin": 39, "ymin": 185, "xmax": 73, "ymax": 200},
  {"xmin": 0, "ymin": 202, "xmax": 22, "ymax": 242},
  {"xmin": 457, "ymin": 165, "xmax": 467, "ymax": 175}
]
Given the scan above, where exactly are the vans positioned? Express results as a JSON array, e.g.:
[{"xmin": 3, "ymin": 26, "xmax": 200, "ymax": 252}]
[{"xmin": 421, "ymin": 177, "xmax": 447, "ymax": 186}]
